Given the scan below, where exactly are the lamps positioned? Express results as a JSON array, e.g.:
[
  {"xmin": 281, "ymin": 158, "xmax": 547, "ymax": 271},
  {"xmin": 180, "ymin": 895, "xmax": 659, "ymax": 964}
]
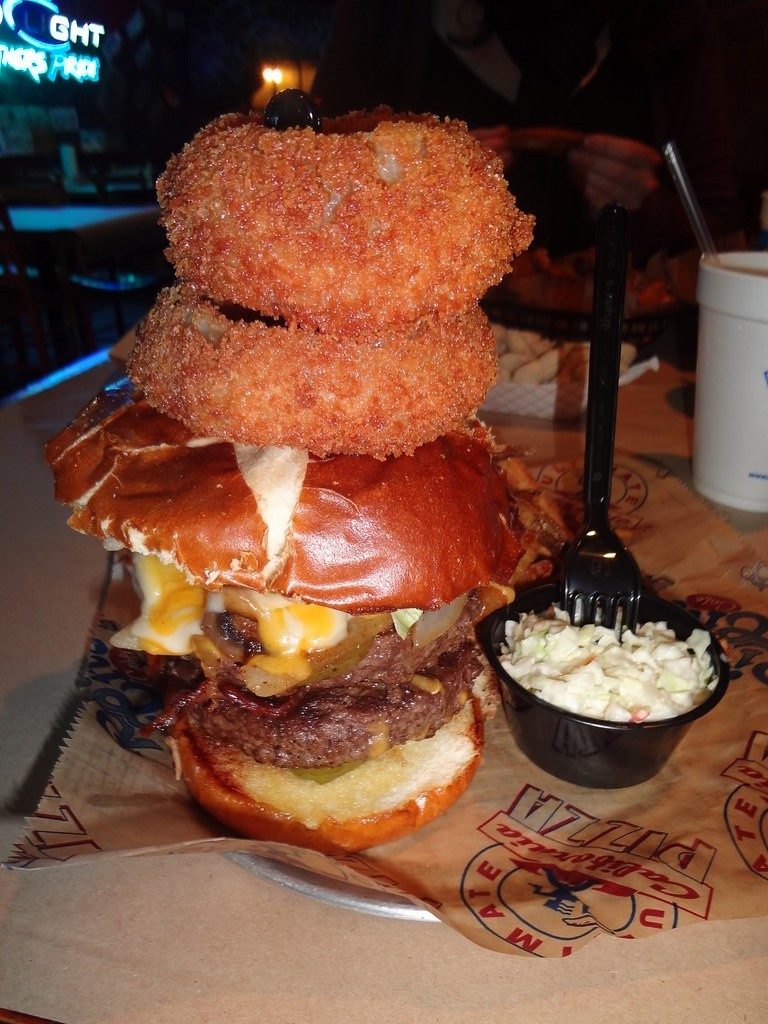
[{"xmin": 255, "ymin": 39, "xmax": 306, "ymax": 111}]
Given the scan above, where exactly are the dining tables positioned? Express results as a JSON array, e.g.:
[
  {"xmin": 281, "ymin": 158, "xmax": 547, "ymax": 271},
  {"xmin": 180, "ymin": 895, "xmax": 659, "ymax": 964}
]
[{"xmin": 0, "ymin": 318, "xmax": 768, "ymax": 1024}]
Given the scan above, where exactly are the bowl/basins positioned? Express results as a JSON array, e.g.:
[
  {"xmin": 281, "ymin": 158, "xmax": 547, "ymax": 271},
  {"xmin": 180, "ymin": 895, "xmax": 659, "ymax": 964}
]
[{"xmin": 478, "ymin": 584, "xmax": 730, "ymax": 787}]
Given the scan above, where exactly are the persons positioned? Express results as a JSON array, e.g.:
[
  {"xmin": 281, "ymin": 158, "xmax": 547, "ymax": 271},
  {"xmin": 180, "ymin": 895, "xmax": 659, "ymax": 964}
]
[
  {"xmin": 153, "ymin": 82, "xmax": 201, "ymax": 145},
  {"xmin": 382, "ymin": 0, "xmax": 663, "ymax": 281}
]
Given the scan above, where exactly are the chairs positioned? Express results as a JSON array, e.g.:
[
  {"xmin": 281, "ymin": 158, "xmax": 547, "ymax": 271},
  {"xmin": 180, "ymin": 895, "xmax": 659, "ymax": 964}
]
[
  {"xmin": 69, "ymin": 234, "xmax": 177, "ymax": 347},
  {"xmin": 457, "ymin": 123, "xmax": 702, "ymax": 341},
  {"xmin": 0, "ymin": 199, "xmax": 50, "ymax": 399}
]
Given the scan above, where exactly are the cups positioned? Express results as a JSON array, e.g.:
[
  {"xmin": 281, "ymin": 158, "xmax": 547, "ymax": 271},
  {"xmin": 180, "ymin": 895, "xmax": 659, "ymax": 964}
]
[{"xmin": 694, "ymin": 251, "xmax": 767, "ymax": 512}]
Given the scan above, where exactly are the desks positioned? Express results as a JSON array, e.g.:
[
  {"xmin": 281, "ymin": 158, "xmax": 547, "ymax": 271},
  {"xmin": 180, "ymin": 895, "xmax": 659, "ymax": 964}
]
[{"xmin": 0, "ymin": 204, "xmax": 163, "ymax": 270}]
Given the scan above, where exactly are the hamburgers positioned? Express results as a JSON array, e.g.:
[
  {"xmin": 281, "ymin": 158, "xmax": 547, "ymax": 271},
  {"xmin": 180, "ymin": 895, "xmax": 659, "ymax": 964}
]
[{"xmin": 50, "ymin": 371, "xmax": 520, "ymax": 854}]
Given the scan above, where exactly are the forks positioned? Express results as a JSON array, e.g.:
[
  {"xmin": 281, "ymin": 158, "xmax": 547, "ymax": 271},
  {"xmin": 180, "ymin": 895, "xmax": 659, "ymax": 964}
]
[{"xmin": 559, "ymin": 202, "xmax": 640, "ymax": 637}]
